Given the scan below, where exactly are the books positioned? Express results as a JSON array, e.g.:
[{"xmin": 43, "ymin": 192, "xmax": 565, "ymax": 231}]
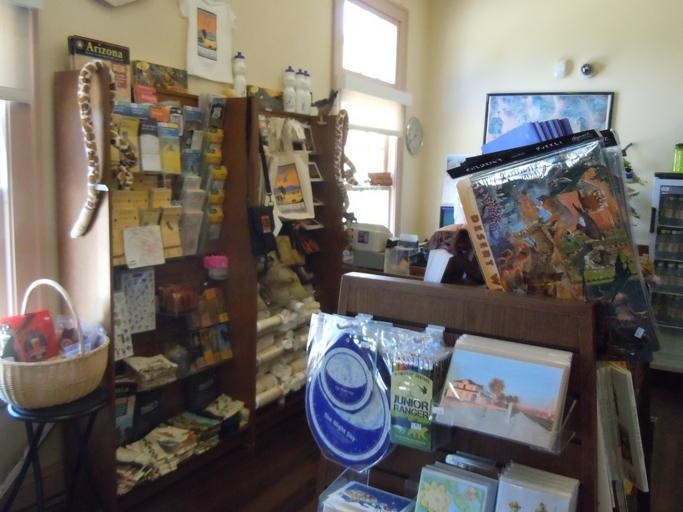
[
  {"xmin": 481, "ymin": 117, "xmax": 574, "ymax": 155},
  {"xmin": 113, "ymin": 396, "xmax": 249, "ymax": 495},
  {"xmin": 107, "ymin": 352, "xmax": 252, "ymax": 496},
  {"xmin": 415, "ymin": 451, "xmax": 582, "ymax": 511},
  {"xmin": 595, "ymin": 361, "xmax": 652, "ymax": 512},
  {"xmin": 114, "ymin": 322, "xmax": 234, "ymax": 393},
  {"xmin": 436, "ymin": 334, "xmax": 573, "ymax": 451},
  {"xmin": 322, "ymin": 482, "xmax": 415, "ymax": 511}
]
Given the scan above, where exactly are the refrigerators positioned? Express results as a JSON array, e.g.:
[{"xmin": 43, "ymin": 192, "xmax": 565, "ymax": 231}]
[{"xmin": 647, "ymin": 171, "xmax": 683, "ymax": 333}]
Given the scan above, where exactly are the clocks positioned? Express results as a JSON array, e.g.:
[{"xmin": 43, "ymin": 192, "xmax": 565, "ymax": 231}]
[{"xmin": 405, "ymin": 117, "xmax": 423, "ymax": 155}]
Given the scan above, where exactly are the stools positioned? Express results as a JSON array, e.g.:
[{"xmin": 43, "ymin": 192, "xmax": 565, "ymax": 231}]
[{"xmin": 2, "ymin": 390, "xmax": 111, "ymax": 511}]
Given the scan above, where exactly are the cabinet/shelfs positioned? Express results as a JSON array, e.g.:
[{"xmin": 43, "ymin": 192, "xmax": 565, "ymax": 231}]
[{"xmin": 57, "ymin": 71, "xmax": 344, "ymax": 511}]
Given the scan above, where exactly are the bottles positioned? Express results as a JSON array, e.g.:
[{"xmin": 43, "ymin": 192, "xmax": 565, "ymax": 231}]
[
  {"xmin": 650, "ymin": 194, "xmax": 683, "ymax": 326},
  {"xmin": 672, "ymin": 143, "xmax": 683, "ymax": 173},
  {"xmin": 232, "ymin": 50, "xmax": 248, "ymax": 98},
  {"xmin": 282, "ymin": 66, "xmax": 312, "ymax": 115}
]
